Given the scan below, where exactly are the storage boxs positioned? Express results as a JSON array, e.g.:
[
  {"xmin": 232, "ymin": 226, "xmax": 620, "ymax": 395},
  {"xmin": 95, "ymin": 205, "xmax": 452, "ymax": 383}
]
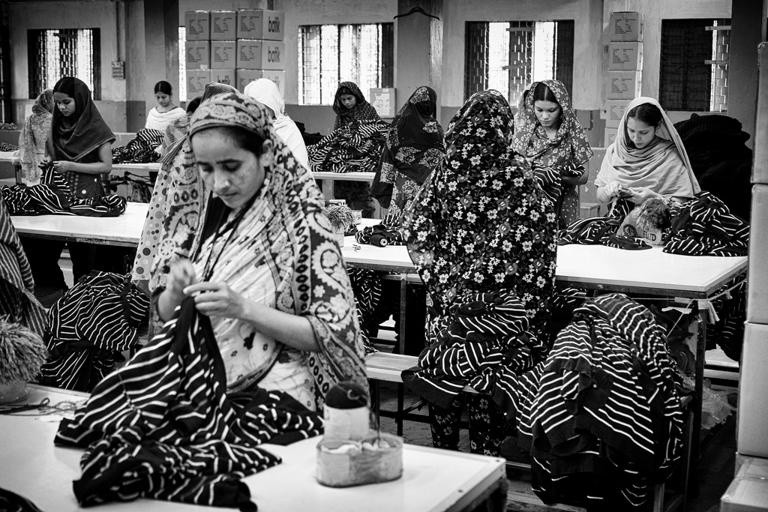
[
  {"xmin": 602, "ymin": 9, "xmax": 646, "ymax": 150},
  {"xmin": 184, "ymin": 8, "xmax": 286, "ymax": 113}
]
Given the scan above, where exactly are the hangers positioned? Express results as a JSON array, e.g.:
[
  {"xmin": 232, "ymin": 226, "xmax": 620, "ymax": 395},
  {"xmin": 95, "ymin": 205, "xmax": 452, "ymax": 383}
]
[{"xmin": 393, "ymin": 1, "xmax": 441, "ymax": 20}]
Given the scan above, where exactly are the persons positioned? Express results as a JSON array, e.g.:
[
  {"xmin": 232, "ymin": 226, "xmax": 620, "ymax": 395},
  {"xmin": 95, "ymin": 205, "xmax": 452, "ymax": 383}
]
[
  {"xmin": 143, "ymin": 90, "xmax": 370, "ymax": 419},
  {"xmin": 409, "ymin": 90, "xmax": 557, "ymax": 455},
  {"xmin": 0, "ymin": 76, "xmax": 444, "ymax": 358},
  {"xmin": 509, "ymin": 80, "xmax": 591, "ymax": 230},
  {"xmin": 596, "ymin": 96, "xmax": 701, "ymax": 208}
]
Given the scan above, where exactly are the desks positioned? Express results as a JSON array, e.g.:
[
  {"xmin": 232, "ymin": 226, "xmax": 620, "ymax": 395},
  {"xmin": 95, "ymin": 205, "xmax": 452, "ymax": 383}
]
[{"xmin": 0, "ymin": 149, "xmax": 749, "ymax": 512}]
